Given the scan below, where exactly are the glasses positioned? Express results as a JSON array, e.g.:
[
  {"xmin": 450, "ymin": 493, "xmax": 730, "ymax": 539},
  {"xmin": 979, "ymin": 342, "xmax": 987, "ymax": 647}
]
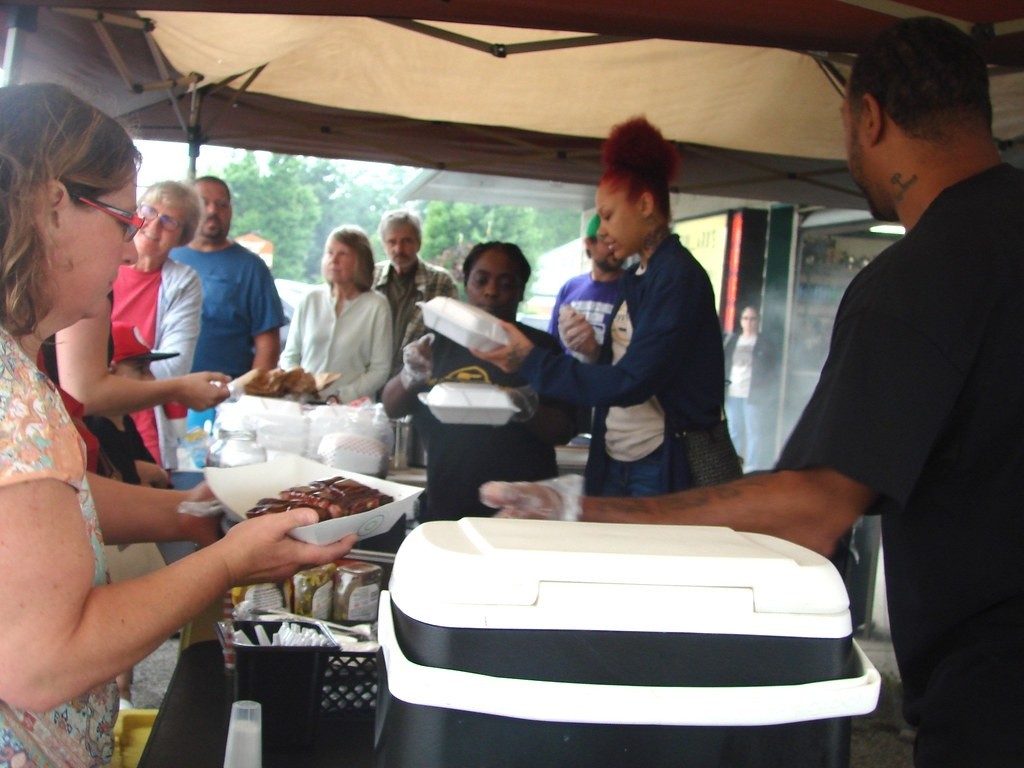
[
  {"xmin": 69, "ymin": 189, "xmax": 148, "ymax": 242},
  {"xmin": 137, "ymin": 205, "xmax": 182, "ymax": 233},
  {"xmin": 741, "ymin": 315, "xmax": 757, "ymax": 320}
]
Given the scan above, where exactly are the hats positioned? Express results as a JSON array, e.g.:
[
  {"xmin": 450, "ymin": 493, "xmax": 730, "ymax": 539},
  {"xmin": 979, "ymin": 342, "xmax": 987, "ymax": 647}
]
[
  {"xmin": 110, "ymin": 322, "xmax": 179, "ymax": 364},
  {"xmin": 587, "ymin": 213, "xmax": 607, "ymax": 240}
]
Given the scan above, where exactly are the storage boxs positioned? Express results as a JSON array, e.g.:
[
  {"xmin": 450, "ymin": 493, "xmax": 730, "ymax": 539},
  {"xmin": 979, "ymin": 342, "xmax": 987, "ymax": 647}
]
[{"xmin": 200, "ymin": 293, "xmax": 882, "ymax": 767}]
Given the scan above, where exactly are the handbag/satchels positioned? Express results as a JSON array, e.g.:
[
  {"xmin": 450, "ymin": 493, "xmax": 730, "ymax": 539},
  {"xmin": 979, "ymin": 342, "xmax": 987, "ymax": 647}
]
[{"xmin": 681, "ymin": 418, "xmax": 744, "ymax": 488}]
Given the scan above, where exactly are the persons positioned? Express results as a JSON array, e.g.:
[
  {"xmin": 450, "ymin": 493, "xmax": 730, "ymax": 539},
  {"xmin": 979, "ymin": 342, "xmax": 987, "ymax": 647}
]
[
  {"xmin": 722, "ymin": 305, "xmax": 775, "ymax": 472},
  {"xmin": 382, "ymin": 239, "xmax": 580, "ymax": 522},
  {"xmin": 36, "ymin": 289, "xmax": 230, "ymax": 489},
  {"xmin": 469, "ymin": 117, "xmax": 744, "ymax": 497},
  {"xmin": 0, "ymin": 83, "xmax": 358, "ymax": 768},
  {"xmin": 478, "ymin": 16, "xmax": 1024, "ymax": 768},
  {"xmin": 547, "ymin": 214, "xmax": 629, "ymax": 365},
  {"xmin": 169, "ymin": 176, "xmax": 289, "ymax": 383},
  {"xmin": 278, "ymin": 225, "xmax": 393, "ymax": 405},
  {"xmin": 112, "ymin": 180, "xmax": 203, "ymax": 476},
  {"xmin": 371, "ymin": 212, "xmax": 459, "ymax": 403}
]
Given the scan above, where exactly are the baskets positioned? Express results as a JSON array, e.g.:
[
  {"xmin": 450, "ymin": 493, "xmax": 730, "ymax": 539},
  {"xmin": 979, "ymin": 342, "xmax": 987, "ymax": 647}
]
[{"xmin": 230, "ymin": 642, "xmax": 381, "ymax": 714}]
